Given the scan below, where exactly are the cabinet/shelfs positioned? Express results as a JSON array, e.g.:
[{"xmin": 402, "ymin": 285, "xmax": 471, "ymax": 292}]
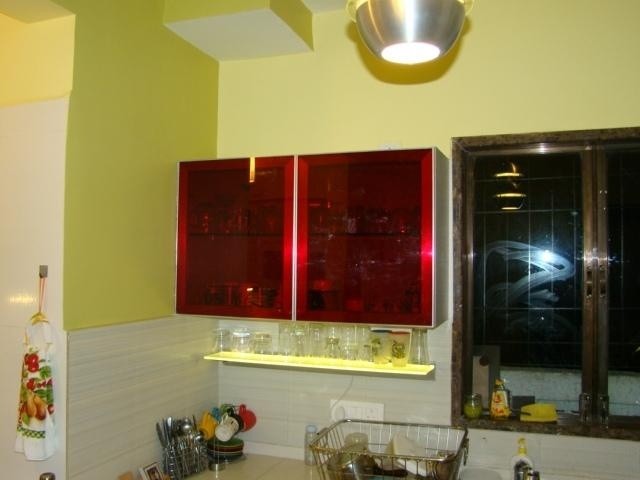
[{"xmin": 175, "ymin": 149, "xmax": 449, "ymax": 330}]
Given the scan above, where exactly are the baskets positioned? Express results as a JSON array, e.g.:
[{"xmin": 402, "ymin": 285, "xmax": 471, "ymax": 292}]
[
  {"xmin": 163, "ymin": 440, "xmax": 209, "ymax": 479},
  {"xmin": 309, "ymin": 420, "xmax": 467, "ymax": 479}
]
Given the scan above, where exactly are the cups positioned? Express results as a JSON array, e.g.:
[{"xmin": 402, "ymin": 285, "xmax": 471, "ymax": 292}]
[
  {"xmin": 579, "ymin": 394, "xmax": 594, "ymax": 428},
  {"xmin": 464, "ymin": 392, "xmax": 482, "ymax": 420},
  {"xmin": 211, "ymin": 323, "xmax": 410, "ymax": 369},
  {"xmin": 198, "ymin": 404, "xmax": 257, "ymax": 442},
  {"xmin": 596, "ymin": 394, "xmax": 609, "ymax": 430}
]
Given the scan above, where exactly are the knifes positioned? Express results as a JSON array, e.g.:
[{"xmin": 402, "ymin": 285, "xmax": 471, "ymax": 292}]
[{"xmin": 156, "ymin": 416, "xmax": 182, "ymax": 479}]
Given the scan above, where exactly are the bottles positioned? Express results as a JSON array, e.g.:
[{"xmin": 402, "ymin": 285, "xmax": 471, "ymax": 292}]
[
  {"xmin": 489, "ymin": 379, "xmax": 509, "ymax": 421},
  {"xmin": 407, "ymin": 328, "xmax": 428, "ymax": 372},
  {"xmin": 303, "ymin": 425, "xmax": 319, "ymax": 467}
]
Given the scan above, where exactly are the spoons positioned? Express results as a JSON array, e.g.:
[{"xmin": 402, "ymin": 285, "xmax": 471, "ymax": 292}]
[{"xmin": 181, "ymin": 416, "xmax": 203, "ymax": 472}]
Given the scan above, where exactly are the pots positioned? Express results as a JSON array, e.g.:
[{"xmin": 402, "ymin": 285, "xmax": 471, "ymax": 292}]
[{"xmin": 327, "ymin": 444, "xmax": 409, "ymax": 479}]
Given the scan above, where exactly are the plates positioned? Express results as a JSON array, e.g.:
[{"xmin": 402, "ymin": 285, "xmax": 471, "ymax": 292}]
[{"xmin": 207, "ymin": 439, "xmax": 245, "ymax": 461}]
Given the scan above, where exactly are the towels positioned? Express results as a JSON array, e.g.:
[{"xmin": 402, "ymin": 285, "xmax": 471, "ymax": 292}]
[{"xmin": 13, "ymin": 344, "xmax": 61, "ymax": 462}]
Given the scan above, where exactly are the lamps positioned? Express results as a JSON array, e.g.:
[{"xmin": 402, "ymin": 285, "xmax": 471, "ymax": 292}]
[
  {"xmin": 345, "ymin": 0, "xmax": 475, "ymax": 66},
  {"xmin": 493, "ymin": 157, "xmax": 527, "ymax": 212}
]
